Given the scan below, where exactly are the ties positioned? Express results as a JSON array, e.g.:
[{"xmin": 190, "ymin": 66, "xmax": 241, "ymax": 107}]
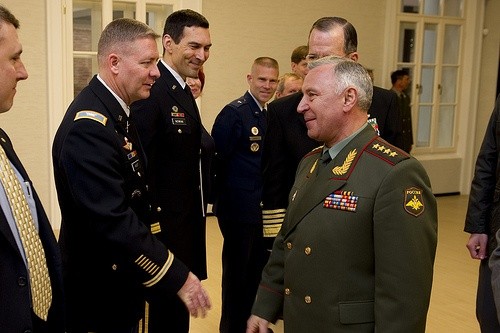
[{"xmin": 0, "ymin": 145, "xmax": 52, "ymax": 322}]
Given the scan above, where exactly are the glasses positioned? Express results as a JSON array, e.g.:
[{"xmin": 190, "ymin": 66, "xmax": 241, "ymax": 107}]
[{"xmin": 305, "ymin": 53, "xmax": 328, "ymax": 66}]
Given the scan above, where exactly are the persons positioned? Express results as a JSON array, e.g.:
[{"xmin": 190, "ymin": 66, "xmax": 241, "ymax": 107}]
[
  {"xmin": 185, "ymin": 66, "xmax": 205, "ymax": 98},
  {"xmin": 130, "ymin": 8, "xmax": 211, "ymax": 333},
  {"xmin": 464, "ymin": 93, "xmax": 500, "ymax": 333},
  {"xmin": 0, "ymin": 5, "xmax": 63, "ymax": 333},
  {"xmin": 274, "ymin": 72, "xmax": 306, "ymax": 100},
  {"xmin": 261, "ymin": 17, "xmax": 404, "ymax": 263},
  {"xmin": 247, "ymin": 56, "xmax": 437, "ymax": 333},
  {"xmin": 52, "ymin": 17, "xmax": 212, "ymax": 333},
  {"xmin": 210, "ymin": 57, "xmax": 280, "ymax": 333},
  {"xmin": 291, "ymin": 45, "xmax": 309, "ymax": 79},
  {"xmin": 390, "ymin": 70, "xmax": 414, "ymax": 154}
]
